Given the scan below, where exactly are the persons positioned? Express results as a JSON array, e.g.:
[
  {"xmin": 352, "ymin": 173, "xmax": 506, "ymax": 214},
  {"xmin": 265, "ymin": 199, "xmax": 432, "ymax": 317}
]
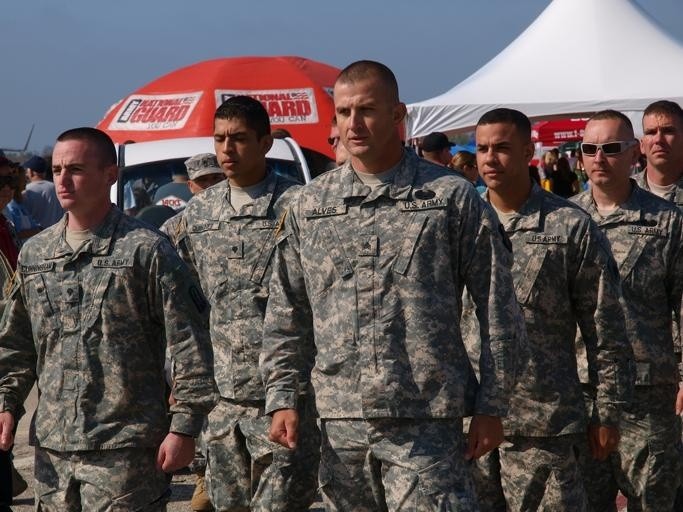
[
  {"xmin": 0, "ymin": 151, "xmax": 65, "ymax": 271},
  {"xmin": 530, "ymin": 148, "xmax": 587, "ymax": 198},
  {"xmin": 259, "ymin": 61, "xmax": 531, "ymax": 512},
  {"xmin": 173, "ymin": 96, "xmax": 321, "ymax": 511},
  {"xmin": 1, "ymin": 446, "xmax": 19, "ymax": 512},
  {"xmin": 628, "ymin": 101, "xmax": 682, "ymax": 380},
  {"xmin": 459, "ymin": 108, "xmax": 637, "ymax": 510},
  {"xmin": 124, "ymin": 153, "xmax": 224, "ymax": 229},
  {"xmin": 408, "ymin": 133, "xmax": 485, "ymax": 195},
  {"xmin": 564, "ymin": 108, "xmax": 683, "ymax": 511},
  {"xmin": 0, "ymin": 129, "xmax": 218, "ymax": 511}
]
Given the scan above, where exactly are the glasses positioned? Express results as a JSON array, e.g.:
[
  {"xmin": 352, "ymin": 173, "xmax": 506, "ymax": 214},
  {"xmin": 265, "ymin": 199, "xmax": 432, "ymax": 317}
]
[{"xmin": 579, "ymin": 140, "xmax": 638, "ymax": 156}]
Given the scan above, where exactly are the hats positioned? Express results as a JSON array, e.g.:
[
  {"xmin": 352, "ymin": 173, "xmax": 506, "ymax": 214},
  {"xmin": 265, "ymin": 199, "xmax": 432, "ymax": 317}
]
[{"xmin": 422, "ymin": 132, "xmax": 456, "ymax": 150}]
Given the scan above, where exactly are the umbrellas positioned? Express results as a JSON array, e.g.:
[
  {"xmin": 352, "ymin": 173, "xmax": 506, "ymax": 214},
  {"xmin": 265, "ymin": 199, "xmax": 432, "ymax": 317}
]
[
  {"xmin": 93, "ymin": 57, "xmax": 404, "ymax": 162},
  {"xmin": 532, "ymin": 117, "xmax": 590, "ymax": 146}
]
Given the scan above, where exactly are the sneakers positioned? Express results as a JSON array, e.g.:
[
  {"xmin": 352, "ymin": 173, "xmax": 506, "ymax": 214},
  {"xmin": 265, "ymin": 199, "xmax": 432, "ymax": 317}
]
[{"xmin": 190, "ymin": 475, "xmax": 213, "ymax": 510}]
